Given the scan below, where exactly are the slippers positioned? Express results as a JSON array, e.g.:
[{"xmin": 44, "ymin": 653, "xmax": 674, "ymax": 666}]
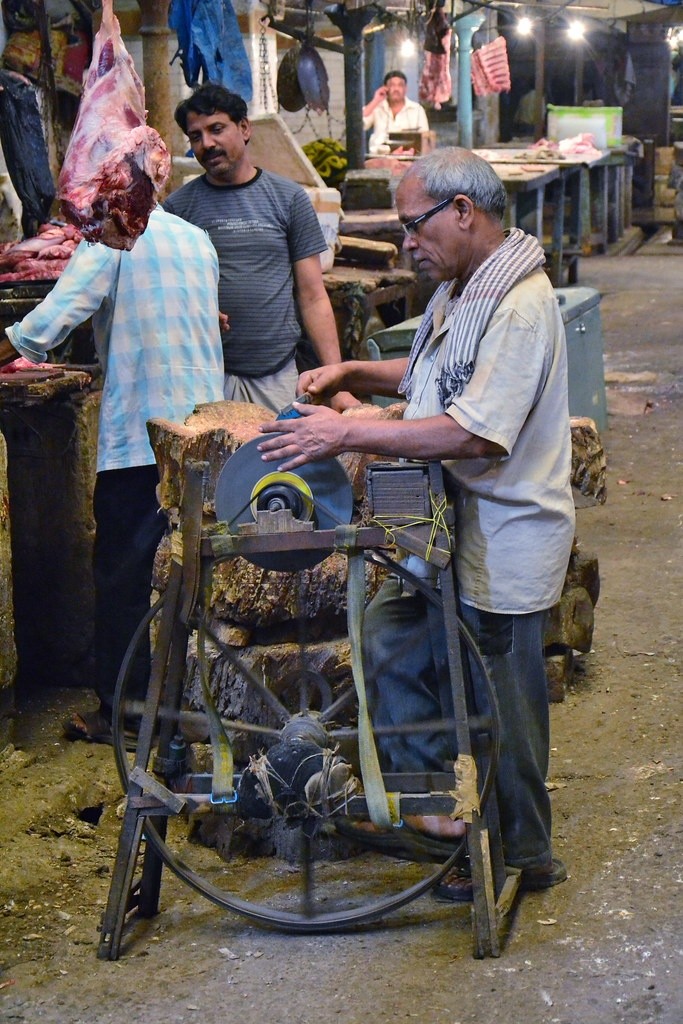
[
  {"xmin": 431, "ymin": 854, "xmax": 567, "ymax": 901},
  {"xmin": 334, "ymin": 808, "xmax": 465, "ymax": 858},
  {"xmin": 59, "ymin": 708, "xmax": 153, "ymax": 753}
]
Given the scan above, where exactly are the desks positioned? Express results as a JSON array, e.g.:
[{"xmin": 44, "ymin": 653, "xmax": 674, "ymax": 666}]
[{"xmin": 471, "ymin": 134, "xmax": 643, "ymax": 286}]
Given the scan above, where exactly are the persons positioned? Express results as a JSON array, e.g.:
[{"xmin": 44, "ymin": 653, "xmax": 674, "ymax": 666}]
[
  {"xmin": 0, "ymin": 198, "xmax": 225, "ymax": 751},
  {"xmin": 257, "ymin": 145, "xmax": 579, "ymax": 903},
  {"xmin": 157, "ymin": 83, "xmax": 375, "ymax": 419},
  {"xmin": 363, "ymin": 69, "xmax": 431, "ymax": 158}
]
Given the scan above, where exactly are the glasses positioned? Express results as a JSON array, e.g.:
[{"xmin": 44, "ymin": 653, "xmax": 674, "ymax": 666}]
[{"xmin": 400, "ymin": 194, "xmax": 458, "ymax": 239}]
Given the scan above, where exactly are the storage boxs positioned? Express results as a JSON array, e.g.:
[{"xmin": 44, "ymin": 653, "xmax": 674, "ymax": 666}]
[
  {"xmin": 558, "ymin": 115, "xmax": 609, "ymax": 147},
  {"xmin": 547, "ymin": 102, "xmax": 624, "ymax": 144},
  {"xmin": 181, "ymin": 174, "xmax": 342, "ymax": 271}
]
